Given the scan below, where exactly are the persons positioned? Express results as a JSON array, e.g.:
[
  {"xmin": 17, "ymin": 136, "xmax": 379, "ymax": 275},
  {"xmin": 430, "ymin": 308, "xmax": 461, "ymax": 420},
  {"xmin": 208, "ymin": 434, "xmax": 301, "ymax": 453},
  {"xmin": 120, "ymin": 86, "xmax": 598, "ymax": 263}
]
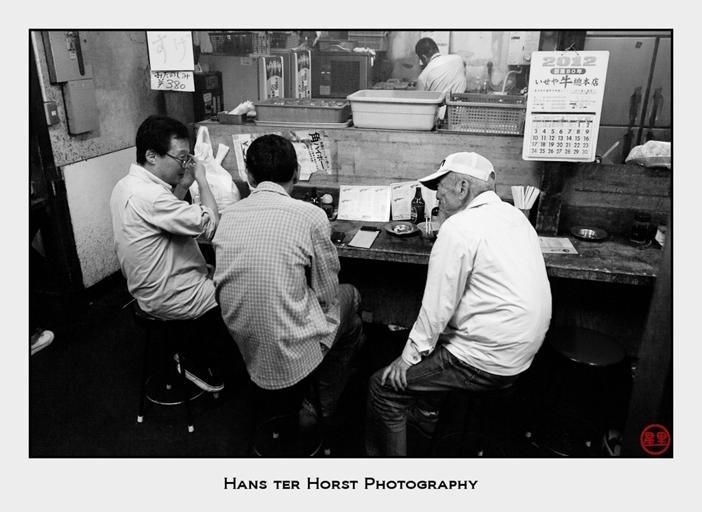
[
  {"xmin": 108, "ymin": 114, "xmax": 227, "ymax": 392},
  {"xmin": 209, "ymin": 134, "xmax": 366, "ymax": 457},
  {"xmin": 368, "ymin": 149, "xmax": 553, "ymax": 458},
  {"xmin": 415, "ymin": 38, "xmax": 468, "ymax": 128}
]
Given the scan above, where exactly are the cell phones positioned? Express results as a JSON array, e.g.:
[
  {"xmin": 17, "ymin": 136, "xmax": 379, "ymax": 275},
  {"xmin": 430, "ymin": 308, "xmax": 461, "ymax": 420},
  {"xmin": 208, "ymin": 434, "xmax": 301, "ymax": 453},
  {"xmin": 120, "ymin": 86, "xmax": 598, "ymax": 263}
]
[{"xmin": 331, "ymin": 232, "xmax": 345, "ymax": 244}]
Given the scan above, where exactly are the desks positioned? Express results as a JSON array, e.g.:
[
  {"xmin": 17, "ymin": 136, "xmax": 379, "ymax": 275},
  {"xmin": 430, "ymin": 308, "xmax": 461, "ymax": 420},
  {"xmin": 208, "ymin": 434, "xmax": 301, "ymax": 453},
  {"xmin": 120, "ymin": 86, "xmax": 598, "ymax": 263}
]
[{"xmin": 193, "ymin": 199, "xmax": 663, "ymax": 357}]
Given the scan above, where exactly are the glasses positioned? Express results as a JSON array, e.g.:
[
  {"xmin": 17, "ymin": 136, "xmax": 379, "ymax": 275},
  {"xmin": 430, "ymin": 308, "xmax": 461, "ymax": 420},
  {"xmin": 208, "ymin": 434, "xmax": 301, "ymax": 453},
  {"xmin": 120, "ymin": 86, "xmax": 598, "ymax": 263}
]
[{"xmin": 166, "ymin": 151, "xmax": 193, "ymax": 169}]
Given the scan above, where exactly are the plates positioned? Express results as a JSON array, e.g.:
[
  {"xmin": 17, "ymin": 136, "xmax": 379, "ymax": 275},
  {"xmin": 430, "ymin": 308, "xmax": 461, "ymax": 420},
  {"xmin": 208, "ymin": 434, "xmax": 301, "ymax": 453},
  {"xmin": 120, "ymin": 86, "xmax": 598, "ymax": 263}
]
[
  {"xmin": 416, "ymin": 220, "xmax": 441, "ymax": 233},
  {"xmin": 570, "ymin": 224, "xmax": 609, "ymax": 241},
  {"xmin": 382, "ymin": 221, "xmax": 420, "ymax": 236}
]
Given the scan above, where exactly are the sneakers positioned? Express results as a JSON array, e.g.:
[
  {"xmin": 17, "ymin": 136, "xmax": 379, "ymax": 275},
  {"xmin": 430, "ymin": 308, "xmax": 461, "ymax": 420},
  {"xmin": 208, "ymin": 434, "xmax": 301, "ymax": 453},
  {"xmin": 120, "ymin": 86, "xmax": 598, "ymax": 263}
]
[{"xmin": 173, "ymin": 351, "xmax": 225, "ymax": 393}]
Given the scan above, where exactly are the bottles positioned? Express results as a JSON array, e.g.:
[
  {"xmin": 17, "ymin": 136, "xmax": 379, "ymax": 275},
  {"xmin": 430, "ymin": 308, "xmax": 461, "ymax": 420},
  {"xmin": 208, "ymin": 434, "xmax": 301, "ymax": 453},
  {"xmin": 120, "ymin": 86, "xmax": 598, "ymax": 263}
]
[
  {"xmin": 320, "ymin": 194, "xmax": 335, "ymax": 219},
  {"xmin": 628, "ymin": 209, "xmax": 652, "ymax": 247},
  {"xmin": 409, "ymin": 184, "xmax": 425, "ymax": 225}
]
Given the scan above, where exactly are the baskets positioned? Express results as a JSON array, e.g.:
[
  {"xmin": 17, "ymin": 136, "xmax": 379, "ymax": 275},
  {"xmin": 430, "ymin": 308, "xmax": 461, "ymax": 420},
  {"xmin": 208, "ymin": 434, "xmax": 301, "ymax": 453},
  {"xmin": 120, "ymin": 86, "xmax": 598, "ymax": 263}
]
[{"xmin": 446, "ymin": 92, "xmax": 526, "ymax": 136}]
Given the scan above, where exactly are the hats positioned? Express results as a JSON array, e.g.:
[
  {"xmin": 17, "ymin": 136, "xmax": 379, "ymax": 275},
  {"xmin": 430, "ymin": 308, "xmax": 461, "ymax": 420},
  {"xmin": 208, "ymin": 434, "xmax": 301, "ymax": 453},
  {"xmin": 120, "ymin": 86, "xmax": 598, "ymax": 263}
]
[{"xmin": 418, "ymin": 152, "xmax": 496, "ymax": 191}]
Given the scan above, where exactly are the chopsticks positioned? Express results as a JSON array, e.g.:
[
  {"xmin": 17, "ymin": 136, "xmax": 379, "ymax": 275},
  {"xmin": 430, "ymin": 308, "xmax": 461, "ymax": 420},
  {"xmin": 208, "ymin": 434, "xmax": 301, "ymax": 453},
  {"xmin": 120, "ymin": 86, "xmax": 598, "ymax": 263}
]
[{"xmin": 509, "ymin": 183, "xmax": 541, "ymax": 211}]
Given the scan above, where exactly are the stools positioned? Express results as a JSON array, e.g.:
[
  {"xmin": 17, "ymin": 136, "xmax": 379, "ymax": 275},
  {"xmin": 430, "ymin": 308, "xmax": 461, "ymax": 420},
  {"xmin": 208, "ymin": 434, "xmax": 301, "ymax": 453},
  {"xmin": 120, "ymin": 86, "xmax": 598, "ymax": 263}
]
[{"xmin": 136, "ymin": 305, "xmax": 636, "ymax": 457}]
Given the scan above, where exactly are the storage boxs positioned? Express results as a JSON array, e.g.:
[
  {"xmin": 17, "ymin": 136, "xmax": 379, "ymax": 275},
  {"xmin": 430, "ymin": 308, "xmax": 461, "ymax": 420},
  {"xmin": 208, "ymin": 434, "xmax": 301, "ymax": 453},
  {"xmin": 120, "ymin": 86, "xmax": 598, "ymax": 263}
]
[{"xmin": 346, "ymin": 89, "xmax": 446, "ymax": 130}]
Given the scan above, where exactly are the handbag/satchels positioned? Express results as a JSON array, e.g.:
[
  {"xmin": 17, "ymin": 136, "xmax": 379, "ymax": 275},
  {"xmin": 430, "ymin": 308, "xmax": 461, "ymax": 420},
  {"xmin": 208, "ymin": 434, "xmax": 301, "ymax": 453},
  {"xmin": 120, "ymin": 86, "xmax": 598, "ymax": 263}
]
[{"xmin": 188, "ymin": 126, "xmax": 241, "ymax": 214}]
